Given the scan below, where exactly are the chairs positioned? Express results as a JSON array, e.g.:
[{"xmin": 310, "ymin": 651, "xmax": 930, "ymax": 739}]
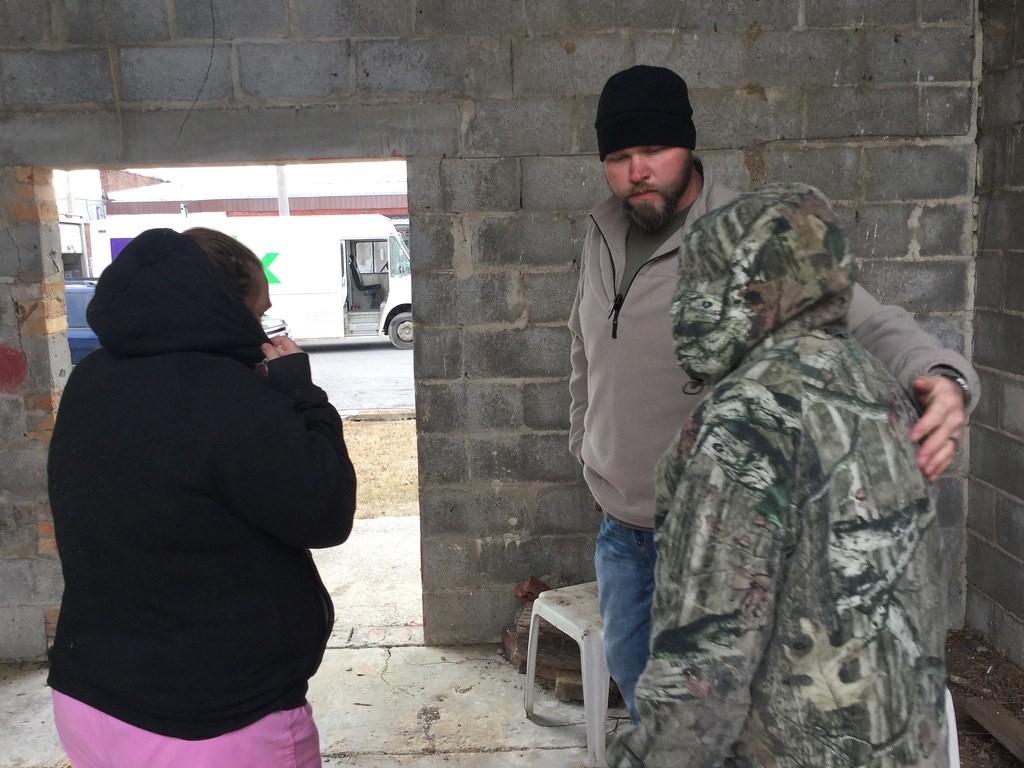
[{"xmin": 349, "ymin": 255, "xmax": 381, "ymax": 291}]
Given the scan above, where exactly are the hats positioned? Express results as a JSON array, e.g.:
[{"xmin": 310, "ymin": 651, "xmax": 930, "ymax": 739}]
[{"xmin": 595, "ymin": 64, "xmax": 696, "ymax": 162}]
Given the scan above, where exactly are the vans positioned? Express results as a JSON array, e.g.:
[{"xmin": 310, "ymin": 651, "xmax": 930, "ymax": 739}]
[{"xmin": 89, "ymin": 214, "xmax": 414, "ymax": 351}]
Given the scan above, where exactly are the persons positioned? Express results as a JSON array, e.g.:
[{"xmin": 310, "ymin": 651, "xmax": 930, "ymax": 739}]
[
  {"xmin": 46, "ymin": 227, "xmax": 357, "ymax": 768},
  {"xmin": 568, "ymin": 65, "xmax": 979, "ymax": 724},
  {"xmin": 597, "ymin": 182, "xmax": 949, "ymax": 768}
]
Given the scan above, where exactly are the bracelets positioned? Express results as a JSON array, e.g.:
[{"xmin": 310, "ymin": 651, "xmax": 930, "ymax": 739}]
[{"xmin": 945, "ymin": 375, "xmax": 971, "ymax": 407}]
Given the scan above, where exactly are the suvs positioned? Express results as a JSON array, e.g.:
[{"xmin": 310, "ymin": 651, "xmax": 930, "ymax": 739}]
[{"xmin": 65, "ymin": 277, "xmax": 293, "ymax": 370}]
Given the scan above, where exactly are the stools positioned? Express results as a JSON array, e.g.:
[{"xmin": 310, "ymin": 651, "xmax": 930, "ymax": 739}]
[{"xmin": 523, "ymin": 581, "xmax": 610, "ymax": 768}]
